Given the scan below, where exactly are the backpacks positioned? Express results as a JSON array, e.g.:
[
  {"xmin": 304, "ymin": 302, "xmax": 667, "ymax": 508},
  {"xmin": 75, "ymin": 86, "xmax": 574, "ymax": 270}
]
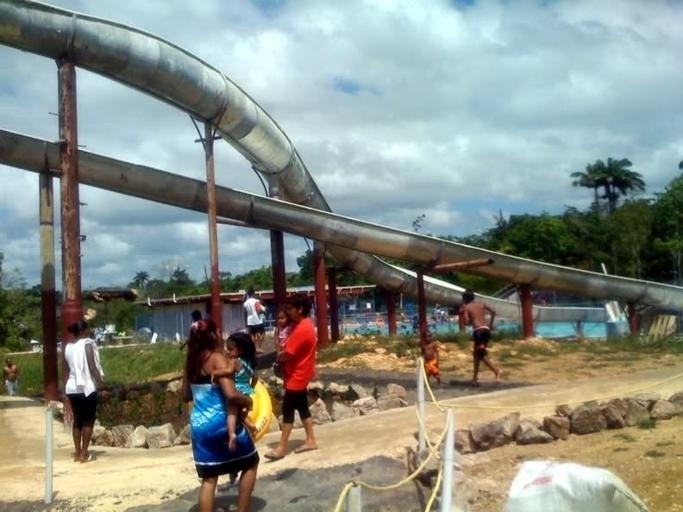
[{"xmin": 239, "ymin": 358, "xmax": 272, "ymax": 441}]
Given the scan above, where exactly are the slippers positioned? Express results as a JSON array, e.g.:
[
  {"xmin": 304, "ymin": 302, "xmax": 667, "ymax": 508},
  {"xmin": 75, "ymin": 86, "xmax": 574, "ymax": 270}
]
[
  {"xmin": 295, "ymin": 441, "xmax": 317, "ymax": 453},
  {"xmin": 81, "ymin": 454, "xmax": 97, "ymax": 463},
  {"xmin": 265, "ymin": 451, "xmax": 285, "ymax": 459}
]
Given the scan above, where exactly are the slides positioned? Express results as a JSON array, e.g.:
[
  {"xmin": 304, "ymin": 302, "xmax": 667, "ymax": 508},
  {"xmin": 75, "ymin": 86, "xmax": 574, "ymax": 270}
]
[{"xmin": 1, "ymin": 0, "xmax": 682, "ymax": 323}]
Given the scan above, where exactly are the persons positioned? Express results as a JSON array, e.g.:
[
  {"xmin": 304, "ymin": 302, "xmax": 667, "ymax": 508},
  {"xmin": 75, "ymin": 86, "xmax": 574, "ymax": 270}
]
[
  {"xmin": 182, "ymin": 320, "xmax": 261, "ymax": 512},
  {"xmin": 263, "ymin": 291, "xmax": 319, "ymax": 460},
  {"xmin": 421, "ymin": 331, "xmax": 441, "ymax": 386},
  {"xmin": 275, "ymin": 304, "xmax": 295, "ymax": 364},
  {"xmin": 413, "ymin": 303, "xmax": 448, "ymax": 333},
  {"xmin": 242, "ymin": 290, "xmax": 262, "ymax": 353},
  {"xmin": 3, "ymin": 357, "xmax": 21, "ymax": 396},
  {"xmin": 179, "ymin": 310, "xmax": 217, "ymax": 351},
  {"xmin": 64, "ymin": 318, "xmax": 107, "ymax": 464},
  {"xmin": 458, "ymin": 288, "xmax": 502, "ymax": 386},
  {"xmin": 255, "ymin": 295, "xmax": 267, "ymax": 354},
  {"xmin": 211, "ymin": 332, "xmax": 258, "ymax": 452}
]
